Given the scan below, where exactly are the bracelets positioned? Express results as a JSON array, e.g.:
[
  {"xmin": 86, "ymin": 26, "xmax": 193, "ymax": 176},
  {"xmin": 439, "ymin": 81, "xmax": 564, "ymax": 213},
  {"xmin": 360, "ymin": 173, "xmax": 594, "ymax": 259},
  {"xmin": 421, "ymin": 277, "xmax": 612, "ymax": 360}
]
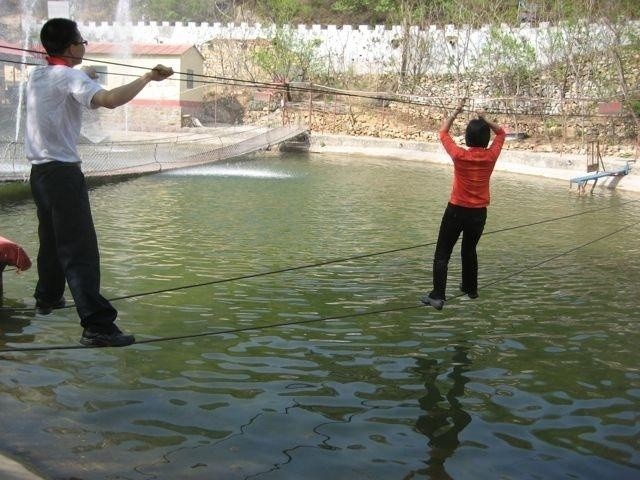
[{"xmin": 450, "ymin": 113, "xmax": 457, "ymax": 120}]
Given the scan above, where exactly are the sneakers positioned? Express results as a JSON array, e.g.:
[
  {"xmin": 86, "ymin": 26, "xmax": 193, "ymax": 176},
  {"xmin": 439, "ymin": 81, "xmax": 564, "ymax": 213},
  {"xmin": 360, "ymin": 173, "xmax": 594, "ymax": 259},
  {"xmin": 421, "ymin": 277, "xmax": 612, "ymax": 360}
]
[
  {"xmin": 80, "ymin": 326, "xmax": 135, "ymax": 348},
  {"xmin": 35, "ymin": 294, "xmax": 66, "ymax": 318},
  {"xmin": 459, "ymin": 282, "xmax": 479, "ymax": 300},
  {"xmin": 421, "ymin": 295, "xmax": 444, "ymax": 311}
]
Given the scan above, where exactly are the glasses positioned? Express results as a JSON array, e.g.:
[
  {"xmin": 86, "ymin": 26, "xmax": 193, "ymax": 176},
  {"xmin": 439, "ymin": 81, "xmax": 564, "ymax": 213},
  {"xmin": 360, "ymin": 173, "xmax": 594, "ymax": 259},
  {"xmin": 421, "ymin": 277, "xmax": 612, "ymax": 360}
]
[{"xmin": 72, "ymin": 39, "xmax": 89, "ymax": 47}]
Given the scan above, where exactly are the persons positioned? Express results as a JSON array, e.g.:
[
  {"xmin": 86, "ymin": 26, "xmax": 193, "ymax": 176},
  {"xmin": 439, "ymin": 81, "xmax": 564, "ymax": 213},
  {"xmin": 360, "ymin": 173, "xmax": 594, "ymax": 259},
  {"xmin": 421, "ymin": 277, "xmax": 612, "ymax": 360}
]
[
  {"xmin": 420, "ymin": 105, "xmax": 506, "ymax": 311},
  {"xmin": 25, "ymin": 18, "xmax": 175, "ymax": 348}
]
